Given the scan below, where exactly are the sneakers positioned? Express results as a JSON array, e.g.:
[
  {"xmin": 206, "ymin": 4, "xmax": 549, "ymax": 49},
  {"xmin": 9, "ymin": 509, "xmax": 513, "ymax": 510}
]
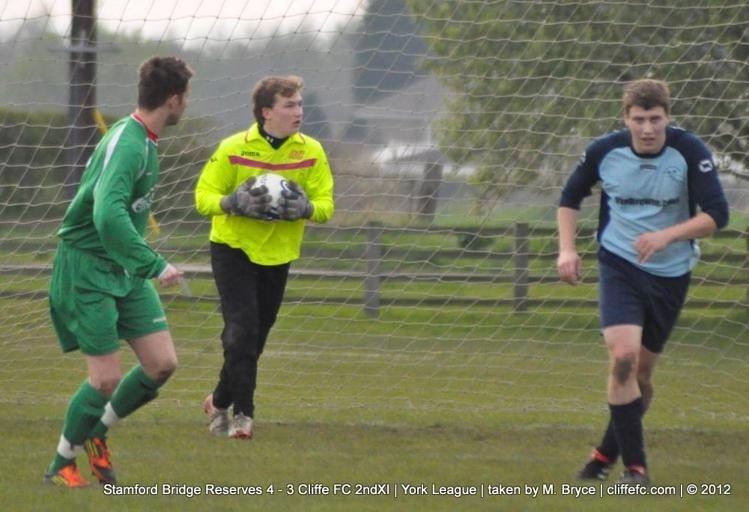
[
  {"xmin": 84, "ymin": 435, "xmax": 116, "ymax": 488},
  {"xmin": 617, "ymin": 468, "xmax": 650, "ymax": 488},
  {"xmin": 579, "ymin": 455, "xmax": 613, "ymax": 484},
  {"xmin": 42, "ymin": 462, "xmax": 91, "ymax": 488},
  {"xmin": 203, "ymin": 394, "xmax": 253, "ymax": 439}
]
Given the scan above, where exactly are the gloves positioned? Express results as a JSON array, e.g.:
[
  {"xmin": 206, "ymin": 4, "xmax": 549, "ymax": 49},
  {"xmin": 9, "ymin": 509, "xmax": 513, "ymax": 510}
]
[
  {"xmin": 277, "ymin": 179, "xmax": 312, "ymax": 221},
  {"xmin": 230, "ymin": 176, "xmax": 270, "ymax": 219}
]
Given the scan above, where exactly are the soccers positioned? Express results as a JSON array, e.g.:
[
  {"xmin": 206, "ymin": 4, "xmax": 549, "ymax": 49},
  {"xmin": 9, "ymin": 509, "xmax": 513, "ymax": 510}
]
[{"xmin": 247, "ymin": 174, "xmax": 292, "ymax": 220}]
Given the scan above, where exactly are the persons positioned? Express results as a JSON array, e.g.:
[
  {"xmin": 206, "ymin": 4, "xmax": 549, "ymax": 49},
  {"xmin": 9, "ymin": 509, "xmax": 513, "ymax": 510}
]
[
  {"xmin": 195, "ymin": 75, "xmax": 336, "ymax": 437},
  {"xmin": 45, "ymin": 55, "xmax": 194, "ymax": 488},
  {"xmin": 556, "ymin": 77, "xmax": 730, "ymax": 484}
]
